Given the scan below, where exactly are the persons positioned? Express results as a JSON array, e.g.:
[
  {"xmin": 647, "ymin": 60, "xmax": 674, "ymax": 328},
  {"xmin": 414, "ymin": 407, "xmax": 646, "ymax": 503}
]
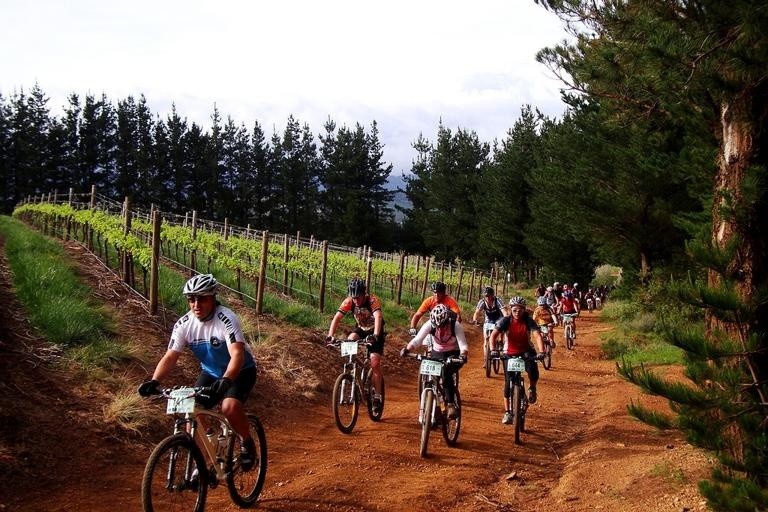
[
  {"xmin": 558, "ymin": 291, "xmax": 579, "ymax": 339},
  {"xmin": 409, "ymin": 282, "xmax": 462, "ymax": 332},
  {"xmin": 585, "ymin": 289, "xmax": 594, "ymax": 309},
  {"xmin": 489, "ymin": 296, "xmax": 546, "ymax": 424},
  {"xmin": 327, "ymin": 279, "xmax": 384, "ymax": 413},
  {"xmin": 401, "ymin": 304, "xmax": 468, "ymax": 427},
  {"xmin": 594, "ymin": 287, "xmax": 603, "ymax": 304},
  {"xmin": 472, "ymin": 286, "xmax": 508, "ymax": 368},
  {"xmin": 562, "ymin": 283, "xmax": 582, "ymax": 310},
  {"xmin": 139, "ymin": 272, "xmax": 256, "ymax": 492},
  {"xmin": 533, "ymin": 297, "xmax": 557, "ymax": 350},
  {"xmin": 535, "ymin": 280, "xmax": 561, "ymax": 324}
]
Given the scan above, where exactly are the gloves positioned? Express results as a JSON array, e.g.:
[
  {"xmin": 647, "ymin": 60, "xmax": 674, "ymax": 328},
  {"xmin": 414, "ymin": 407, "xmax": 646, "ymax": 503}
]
[
  {"xmin": 325, "ymin": 335, "xmax": 334, "ymax": 343},
  {"xmin": 490, "ymin": 348, "xmax": 500, "ymax": 360},
  {"xmin": 399, "ymin": 347, "xmax": 409, "ymax": 359},
  {"xmin": 536, "ymin": 351, "xmax": 545, "ymax": 361},
  {"xmin": 365, "ymin": 334, "xmax": 379, "ymax": 344},
  {"xmin": 138, "ymin": 381, "xmax": 160, "ymax": 396},
  {"xmin": 410, "ymin": 327, "xmax": 420, "ymax": 337},
  {"xmin": 446, "ymin": 354, "xmax": 455, "ymax": 366},
  {"xmin": 205, "ymin": 379, "xmax": 230, "ymax": 406}
]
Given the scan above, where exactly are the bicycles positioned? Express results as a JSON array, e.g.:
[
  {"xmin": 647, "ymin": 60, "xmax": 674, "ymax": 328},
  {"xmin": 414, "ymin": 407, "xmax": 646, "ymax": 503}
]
[
  {"xmin": 587, "ymin": 298, "xmax": 594, "ymax": 314},
  {"xmin": 493, "ymin": 355, "xmax": 544, "ymax": 444},
  {"xmin": 476, "ymin": 321, "xmax": 500, "ymax": 379},
  {"xmin": 560, "ymin": 313, "xmax": 576, "ymax": 350},
  {"xmin": 538, "ymin": 323, "xmax": 554, "ymax": 371},
  {"xmin": 534, "ymin": 295, "xmax": 540, "ymax": 298},
  {"xmin": 141, "ymin": 385, "xmax": 268, "ymax": 512},
  {"xmin": 404, "ymin": 352, "xmax": 463, "ymax": 458},
  {"xmin": 595, "ymin": 297, "xmax": 602, "ymax": 310},
  {"xmin": 326, "ymin": 339, "xmax": 385, "ymax": 434}
]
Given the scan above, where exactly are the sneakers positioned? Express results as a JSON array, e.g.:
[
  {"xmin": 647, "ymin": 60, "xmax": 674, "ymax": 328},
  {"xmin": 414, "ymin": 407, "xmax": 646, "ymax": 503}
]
[
  {"xmin": 501, "ymin": 411, "xmax": 513, "ymax": 425},
  {"xmin": 448, "ymin": 404, "xmax": 458, "ymax": 420},
  {"xmin": 527, "ymin": 386, "xmax": 537, "ymax": 403},
  {"xmin": 372, "ymin": 398, "xmax": 383, "ymax": 412},
  {"xmin": 240, "ymin": 439, "xmax": 257, "ymax": 471}
]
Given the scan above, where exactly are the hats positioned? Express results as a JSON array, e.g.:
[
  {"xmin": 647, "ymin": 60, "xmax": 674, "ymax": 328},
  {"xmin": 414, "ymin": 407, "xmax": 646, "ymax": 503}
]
[
  {"xmin": 574, "ymin": 283, "xmax": 580, "ymax": 288},
  {"xmin": 483, "ymin": 287, "xmax": 495, "ymax": 297},
  {"xmin": 554, "ymin": 282, "xmax": 561, "ymax": 287},
  {"xmin": 547, "ymin": 286, "xmax": 553, "ymax": 291}
]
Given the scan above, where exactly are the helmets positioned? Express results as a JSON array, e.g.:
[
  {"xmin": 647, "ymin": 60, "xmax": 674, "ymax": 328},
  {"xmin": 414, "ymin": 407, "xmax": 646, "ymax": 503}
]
[
  {"xmin": 537, "ymin": 296, "xmax": 546, "ymax": 304},
  {"xmin": 430, "ymin": 282, "xmax": 446, "ymax": 293},
  {"xmin": 430, "ymin": 303, "xmax": 448, "ymax": 328},
  {"xmin": 562, "ymin": 291, "xmax": 571, "ymax": 297},
  {"xmin": 349, "ymin": 277, "xmax": 366, "ymax": 299},
  {"xmin": 509, "ymin": 296, "xmax": 526, "ymax": 309},
  {"xmin": 182, "ymin": 273, "xmax": 219, "ymax": 297}
]
